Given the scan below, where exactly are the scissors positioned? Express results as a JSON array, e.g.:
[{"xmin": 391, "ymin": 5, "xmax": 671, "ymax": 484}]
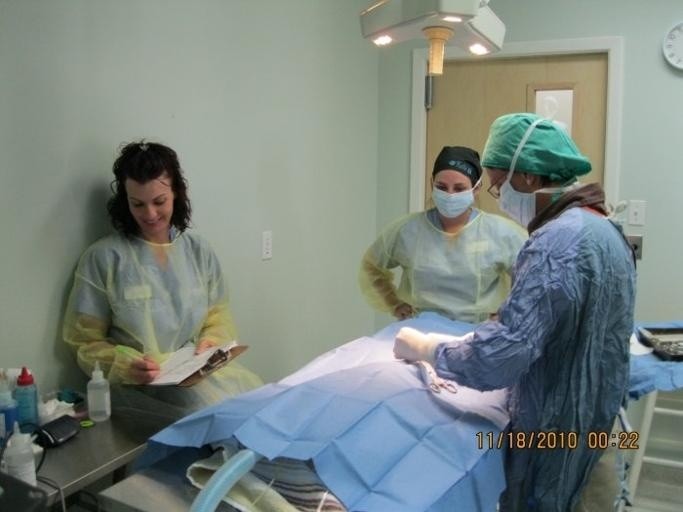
[{"xmin": 429, "ymin": 371, "xmax": 457, "ymax": 394}]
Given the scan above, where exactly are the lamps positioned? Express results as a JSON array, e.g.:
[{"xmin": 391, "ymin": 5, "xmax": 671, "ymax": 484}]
[{"xmin": 359, "ymin": 1, "xmax": 508, "ymax": 77}]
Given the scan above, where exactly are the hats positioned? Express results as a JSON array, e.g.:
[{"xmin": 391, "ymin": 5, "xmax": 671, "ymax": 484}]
[
  {"xmin": 432, "ymin": 146, "xmax": 483, "ymax": 183},
  {"xmin": 481, "ymin": 112, "xmax": 593, "ymax": 182}
]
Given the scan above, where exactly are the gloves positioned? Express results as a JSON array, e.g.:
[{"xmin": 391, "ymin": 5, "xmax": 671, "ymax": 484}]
[{"xmin": 392, "ymin": 326, "xmax": 442, "ymax": 364}]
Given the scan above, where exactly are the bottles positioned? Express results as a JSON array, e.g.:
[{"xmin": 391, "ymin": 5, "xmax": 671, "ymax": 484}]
[
  {"xmin": 3, "ymin": 420, "xmax": 37, "ymax": 490},
  {"xmin": 0, "ymin": 366, "xmax": 40, "ymax": 448},
  {"xmin": 86, "ymin": 360, "xmax": 112, "ymax": 423}
]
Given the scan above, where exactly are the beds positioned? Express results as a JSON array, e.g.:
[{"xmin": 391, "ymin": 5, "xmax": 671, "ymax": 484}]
[{"xmin": 97, "ymin": 313, "xmax": 514, "ymax": 512}]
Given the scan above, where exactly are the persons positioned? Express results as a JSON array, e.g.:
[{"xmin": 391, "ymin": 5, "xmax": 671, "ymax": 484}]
[
  {"xmin": 56, "ymin": 139, "xmax": 266, "ymax": 433},
  {"xmin": 391, "ymin": 111, "xmax": 639, "ymax": 512},
  {"xmin": 356, "ymin": 144, "xmax": 528, "ymax": 327}
]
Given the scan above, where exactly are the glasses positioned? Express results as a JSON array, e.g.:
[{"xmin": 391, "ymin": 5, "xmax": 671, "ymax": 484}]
[{"xmin": 486, "ymin": 173, "xmax": 506, "ymax": 199}]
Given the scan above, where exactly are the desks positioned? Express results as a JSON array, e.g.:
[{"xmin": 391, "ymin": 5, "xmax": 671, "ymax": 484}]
[{"xmin": 25, "ymin": 404, "xmax": 163, "ymax": 512}]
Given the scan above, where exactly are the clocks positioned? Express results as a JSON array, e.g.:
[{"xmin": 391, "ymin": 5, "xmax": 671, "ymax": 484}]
[{"xmin": 661, "ymin": 22, "xmax": 682, "ymax": 71}]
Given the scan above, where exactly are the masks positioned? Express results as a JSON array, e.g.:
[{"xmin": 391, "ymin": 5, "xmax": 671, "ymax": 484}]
[
  {"xmin": 431, "ymin": 179, "xmax": 481, "ymax": 218},
  {"xmin": 494, "ymin": 158, "xmax": 559, "ymax": 228}
]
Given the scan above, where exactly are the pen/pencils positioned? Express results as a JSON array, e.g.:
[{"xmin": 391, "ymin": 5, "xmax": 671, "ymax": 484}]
[{"xmin": 117, "ymin": 345, "xmax": 142, "ymax": 358}]
